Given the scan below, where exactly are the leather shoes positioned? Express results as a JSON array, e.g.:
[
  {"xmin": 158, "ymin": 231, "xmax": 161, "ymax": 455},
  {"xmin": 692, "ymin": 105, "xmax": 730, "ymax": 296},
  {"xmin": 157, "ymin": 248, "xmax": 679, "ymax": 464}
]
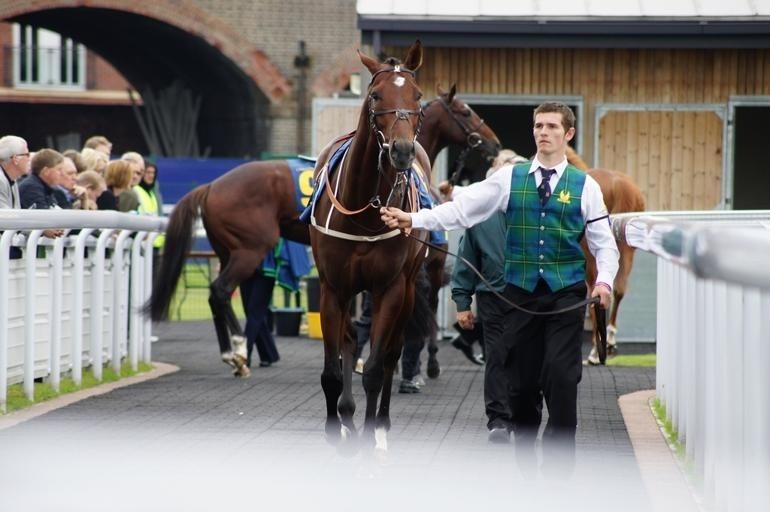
[{"xmin": 451, "ymin": 335, "xmax": 485, "ymax": 365}]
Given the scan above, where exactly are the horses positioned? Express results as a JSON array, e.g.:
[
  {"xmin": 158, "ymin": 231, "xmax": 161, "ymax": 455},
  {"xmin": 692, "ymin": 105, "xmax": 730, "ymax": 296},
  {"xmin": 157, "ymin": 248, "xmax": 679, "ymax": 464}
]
[
  {"xmin": 137, "ymin": 80, "xmax": 503, "ymax": 378},
  {"xmin": 426, "ymin": 190, "xmax": 449, "ymax": 379},
  {"xmin": 308, "ymin": 37, "xmax": 431, "ymax": 463},
  {"xmin": 564, "ymin": 144, "xmax": 646, "ymax": 367}
]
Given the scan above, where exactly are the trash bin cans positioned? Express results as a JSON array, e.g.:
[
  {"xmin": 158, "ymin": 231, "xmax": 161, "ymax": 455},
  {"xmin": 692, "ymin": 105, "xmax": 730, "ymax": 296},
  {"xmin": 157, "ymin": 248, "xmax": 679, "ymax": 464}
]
[{"xmin": 302, "ymin": 266, "xmax": 361, "ymax": 339}]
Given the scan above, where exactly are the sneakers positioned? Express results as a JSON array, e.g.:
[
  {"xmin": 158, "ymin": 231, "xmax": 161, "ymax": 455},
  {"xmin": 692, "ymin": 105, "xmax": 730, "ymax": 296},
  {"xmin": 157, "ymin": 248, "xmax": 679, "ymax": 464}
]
[
  {"xmin": 338, "ymin": 355, "xmax": 365, "ymax": 375},
  {"xmin": 398, "ymin": 381, "xmax": 422, "ymax": 393},
  {"xmin": 245, "ymin": 356, "xmax": 279, "ymax": 368},
  {"xmin": 488, "ymin": 428, "xmax": 511, "ymax": 446}
]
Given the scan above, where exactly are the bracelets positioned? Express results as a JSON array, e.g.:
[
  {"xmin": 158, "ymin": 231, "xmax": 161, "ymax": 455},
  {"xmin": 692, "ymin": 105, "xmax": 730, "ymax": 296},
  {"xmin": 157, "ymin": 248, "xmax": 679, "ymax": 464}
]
[{"xmin": 594, "ymin": 283, "xmax": 612, "ymax": 292}]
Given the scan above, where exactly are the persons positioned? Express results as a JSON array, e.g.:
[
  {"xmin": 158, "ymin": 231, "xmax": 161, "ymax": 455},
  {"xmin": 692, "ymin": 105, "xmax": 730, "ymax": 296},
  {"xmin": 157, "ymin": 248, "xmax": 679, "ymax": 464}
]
[
  {"xmin": 379, "ymin": 100, "xmax": 621, "ymax": 492},
  {"xmin": 452, "ymin": 157, "xmax": 531, "ymax": 462},
  {"xmin": 350, "ymin": 260, "xmax": 431, "ymax": 393},
  {"xmin": 240, "ymin": 269, "xmax": 280, "ymax": 367},
  {"xmin": 0, "ymin": 134, "xmax": 165, "ymax": 342},
  {"xmin": 451, "ymin": 150, "xmax": 515, "ymax": 367}
]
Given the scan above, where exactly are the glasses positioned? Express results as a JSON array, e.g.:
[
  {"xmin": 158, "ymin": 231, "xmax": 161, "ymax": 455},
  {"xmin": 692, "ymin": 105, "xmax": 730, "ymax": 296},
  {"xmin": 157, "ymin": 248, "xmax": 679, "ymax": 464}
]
[{"xmin": 10, "ymin": 153, "xmax": 30, "ymax": 159}]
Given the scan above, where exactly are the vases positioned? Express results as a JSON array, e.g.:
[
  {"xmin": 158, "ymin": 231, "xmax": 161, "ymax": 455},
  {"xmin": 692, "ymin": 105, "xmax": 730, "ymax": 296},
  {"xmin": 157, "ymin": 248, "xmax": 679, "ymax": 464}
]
[
  {"xmin": 273, "ymin": 307, "xmax": 305, "ymax": 338},
  {"xmin": 303, "ymin": 274, "xmax": 358, "ymax": 340}
]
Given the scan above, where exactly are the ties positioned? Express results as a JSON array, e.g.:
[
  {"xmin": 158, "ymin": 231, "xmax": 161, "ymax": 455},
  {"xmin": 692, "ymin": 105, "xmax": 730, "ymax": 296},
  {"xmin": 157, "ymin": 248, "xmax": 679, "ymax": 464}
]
[{"xmin": 537, "ymin": 166, "xmax": 557, "ymax": 207}]
